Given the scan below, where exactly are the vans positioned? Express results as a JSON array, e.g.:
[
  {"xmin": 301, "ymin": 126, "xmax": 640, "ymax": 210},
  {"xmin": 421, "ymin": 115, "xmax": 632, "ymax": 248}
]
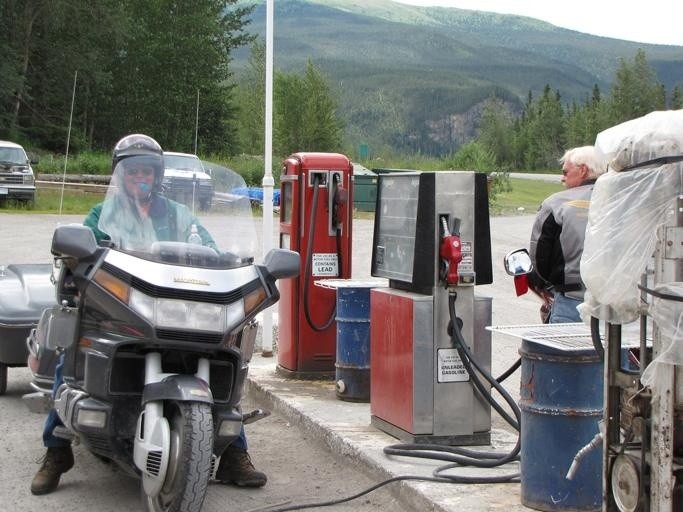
[
  {"xmin": 0, "ymin": 140, "xmax": 37, "ymax": 209},
  {"xmin": 160, "ymin": 151, "xmax": 214, "ymax": 211}
]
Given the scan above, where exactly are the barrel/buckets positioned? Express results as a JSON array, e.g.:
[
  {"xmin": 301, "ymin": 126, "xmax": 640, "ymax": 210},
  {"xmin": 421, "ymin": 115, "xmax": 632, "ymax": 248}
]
[
  {"xmin": 518, "ymin": 337, "xmax": 629, "ymax": 512},
  {"xmin": 335, "ymin": 286, "xmax": 375, "ymax": 404}
]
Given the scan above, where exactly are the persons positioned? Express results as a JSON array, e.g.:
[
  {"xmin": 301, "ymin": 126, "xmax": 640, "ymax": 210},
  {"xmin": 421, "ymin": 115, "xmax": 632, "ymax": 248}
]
[
  {"xmin": 31, "ymin": 132, "xmax": 267, "ymax": 496},
  {"xmin": 531, "ymin": 146, "xmax": 631, "ymax": 373}
]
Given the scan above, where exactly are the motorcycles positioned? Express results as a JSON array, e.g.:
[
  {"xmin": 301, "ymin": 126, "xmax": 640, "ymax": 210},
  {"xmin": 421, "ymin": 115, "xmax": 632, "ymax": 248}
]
[
  {"xmin": 504, "ymin": 247, "xmax": 555, "ymax": 321},
  {"xmin": 27, "ymin": 155, "xmax": 303, "ymax": 512}
]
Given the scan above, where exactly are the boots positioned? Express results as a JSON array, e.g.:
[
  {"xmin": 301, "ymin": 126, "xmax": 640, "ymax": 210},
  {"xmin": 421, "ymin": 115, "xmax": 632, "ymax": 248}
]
[
  {"xmin": 214, "ymin": 445, "xmax": 268, "ymax": 488},
  {"xmin": 30, "ymin": 447, "xmax": 75, "ymax": 495}
]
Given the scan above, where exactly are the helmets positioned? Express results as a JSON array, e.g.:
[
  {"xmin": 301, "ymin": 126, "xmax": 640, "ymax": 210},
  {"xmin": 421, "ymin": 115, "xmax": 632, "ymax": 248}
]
[{"xmin": 113, "ymin": 133, "xmax": 165, "ymax": 193}]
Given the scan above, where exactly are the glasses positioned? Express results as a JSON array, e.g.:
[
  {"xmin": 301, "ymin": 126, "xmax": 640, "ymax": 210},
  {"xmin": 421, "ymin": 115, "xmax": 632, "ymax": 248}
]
[
  {"xmin": 127, "ymin": 167, "xmax": 155, "ymax": 176},
  {"xmin": 561, "ymin": 166, "xmax": 585, "ymax": 176}
]
